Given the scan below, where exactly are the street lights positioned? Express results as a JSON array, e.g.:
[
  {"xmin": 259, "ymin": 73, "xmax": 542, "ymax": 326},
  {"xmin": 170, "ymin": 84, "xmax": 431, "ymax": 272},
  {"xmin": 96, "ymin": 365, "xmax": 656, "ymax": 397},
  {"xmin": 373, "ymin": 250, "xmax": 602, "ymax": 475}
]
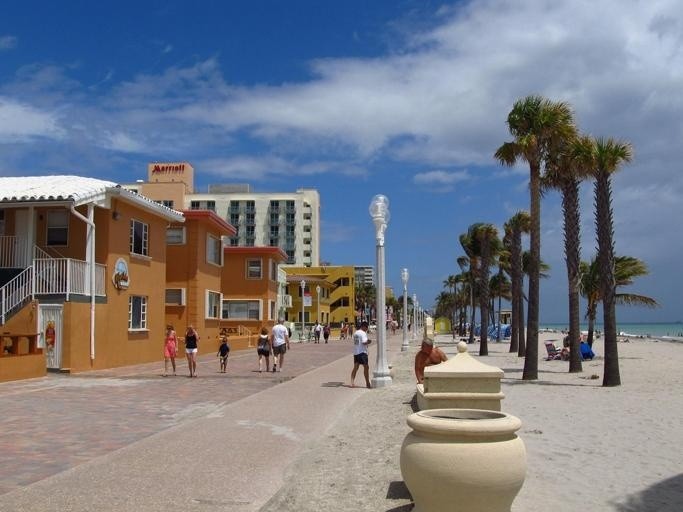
[
  {"xmin": 398, "ymin": 261, "xmax": 412, "ymax": 352},
  {"xmin": 315, "ymin": 285, "xmax": 321, "ymax": 325},
  {"xmin": 299, "ymin": 278, "xmax": 306, "ymax": 345},
  {"xmin": 366, "ymin": 192, "xmax": 397, "ymax": 387},
  {"xmin": 409, "ymin": 290, "xmax": 432, "ymax": 339}
]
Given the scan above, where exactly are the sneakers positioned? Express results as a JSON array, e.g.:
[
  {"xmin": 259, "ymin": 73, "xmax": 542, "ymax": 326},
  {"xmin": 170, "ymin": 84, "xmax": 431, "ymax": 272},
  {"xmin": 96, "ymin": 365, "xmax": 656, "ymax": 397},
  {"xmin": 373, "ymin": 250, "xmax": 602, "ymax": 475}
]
[{"xmin": 273, "ymin": 364, "xmax": 277, "ymax": 372}]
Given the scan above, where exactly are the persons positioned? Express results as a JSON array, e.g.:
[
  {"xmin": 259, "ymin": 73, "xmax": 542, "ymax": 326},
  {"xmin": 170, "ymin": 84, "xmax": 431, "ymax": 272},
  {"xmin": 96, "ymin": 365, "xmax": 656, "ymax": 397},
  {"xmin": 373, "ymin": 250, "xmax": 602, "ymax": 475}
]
[
  {"xmin": 282, "ymin": 318, "xmax": 398, "ymax": 344},
  {"xmin": 256, "ymin": 326, "xmax": 272, "ymax": 373},
  {"xmin": 216, "ymin": 336, "xmax": 230, "ymax": 373},
  {"xmin": 414, "ymin": 338, "xmax": 448, "ymax": 384},
  {"xmin": 560, "ymin": 331, "xmax": 594, "ymax": 361},
  {"xmin": 350, "ymin": 321, "xmax": 374, "ymax": 389},
  {"xmin": 272, "ymin": 317, "xmax": 290, "ymax": 372},
  {"xmin": 162, "ymin": 324, "xmax": 178, "ymax": 377},
  {"xmin": 183, "ymin": 326, "xmax": 198, "ymax": 376}
]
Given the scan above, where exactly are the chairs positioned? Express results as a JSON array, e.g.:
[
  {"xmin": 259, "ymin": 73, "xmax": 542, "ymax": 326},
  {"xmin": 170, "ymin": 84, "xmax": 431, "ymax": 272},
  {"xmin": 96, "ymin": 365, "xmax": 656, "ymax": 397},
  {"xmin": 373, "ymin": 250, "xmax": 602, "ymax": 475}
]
[{"xmin": 544, "ymin": 340, "xmax": 562, "ymax": 358}]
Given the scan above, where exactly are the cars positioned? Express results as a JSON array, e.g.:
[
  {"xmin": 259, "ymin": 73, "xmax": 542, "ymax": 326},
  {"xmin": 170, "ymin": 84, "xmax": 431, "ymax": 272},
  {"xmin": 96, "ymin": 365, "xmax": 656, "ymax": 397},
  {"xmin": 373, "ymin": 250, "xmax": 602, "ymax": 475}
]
[{"xmin": 357, "ymin": 319, "xmax": 411, "ymax": 333}]
